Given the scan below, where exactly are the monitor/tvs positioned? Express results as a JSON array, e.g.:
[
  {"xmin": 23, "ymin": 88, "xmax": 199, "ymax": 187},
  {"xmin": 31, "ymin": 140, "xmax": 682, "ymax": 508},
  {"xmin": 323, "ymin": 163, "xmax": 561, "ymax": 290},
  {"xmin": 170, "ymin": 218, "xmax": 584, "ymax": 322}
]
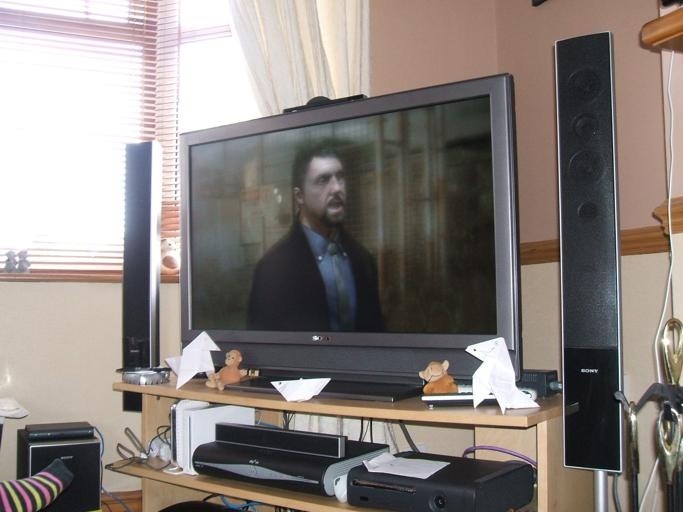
[{"xmin": 180, "ymin": 74, "xmax": 523, "ymax": 402}]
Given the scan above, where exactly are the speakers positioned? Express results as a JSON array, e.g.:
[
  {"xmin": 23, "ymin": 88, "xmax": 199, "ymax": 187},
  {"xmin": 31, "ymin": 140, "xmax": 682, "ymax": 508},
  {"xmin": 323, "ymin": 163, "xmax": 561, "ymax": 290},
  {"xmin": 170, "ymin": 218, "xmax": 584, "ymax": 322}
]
[
  {"xmin": 555, "ymin": 31, "xmax": 623, "ymax": 473},
  {"xmin": 17, "ymin": 429, "xmax": 101, "ymax": 512},
  {"xmin": 123, "ymin": 140, "xmax": 164, "ymax": 412}
]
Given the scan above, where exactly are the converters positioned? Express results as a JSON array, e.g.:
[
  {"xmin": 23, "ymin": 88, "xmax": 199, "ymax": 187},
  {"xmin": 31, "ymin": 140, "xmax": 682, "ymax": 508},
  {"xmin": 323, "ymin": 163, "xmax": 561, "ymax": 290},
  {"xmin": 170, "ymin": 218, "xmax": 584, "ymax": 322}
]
[{"xmin": 517, "ymin": 370, "xmax": 556, "ymax": 399}]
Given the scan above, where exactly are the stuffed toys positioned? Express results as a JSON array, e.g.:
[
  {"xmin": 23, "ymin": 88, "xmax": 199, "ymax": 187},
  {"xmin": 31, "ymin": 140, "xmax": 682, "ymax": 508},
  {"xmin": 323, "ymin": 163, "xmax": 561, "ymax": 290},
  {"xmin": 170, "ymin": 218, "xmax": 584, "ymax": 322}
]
[
  {"xmin": 418, "ymin": 359, "xmax": 458, "ymax": 395},
  {"xmin": 206, "ymin": 349, "xmax": 248, "ymax": 391}
]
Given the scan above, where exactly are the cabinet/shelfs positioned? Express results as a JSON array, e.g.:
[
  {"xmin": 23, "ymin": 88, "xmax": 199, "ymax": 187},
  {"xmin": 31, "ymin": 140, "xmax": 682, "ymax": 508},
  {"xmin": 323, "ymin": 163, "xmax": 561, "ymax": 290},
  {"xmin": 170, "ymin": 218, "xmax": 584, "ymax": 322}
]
[{"xmin": 103, "ymin": 375, "xmax": 594, "ymax": 512}]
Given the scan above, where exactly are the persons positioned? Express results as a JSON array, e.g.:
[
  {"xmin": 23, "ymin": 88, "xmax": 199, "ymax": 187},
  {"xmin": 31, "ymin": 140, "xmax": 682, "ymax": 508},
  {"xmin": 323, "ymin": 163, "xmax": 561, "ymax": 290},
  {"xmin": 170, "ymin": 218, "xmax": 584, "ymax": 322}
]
[{"xmin": 245, "ymin": 147, "xmax": 388, "ymax": 333}]
